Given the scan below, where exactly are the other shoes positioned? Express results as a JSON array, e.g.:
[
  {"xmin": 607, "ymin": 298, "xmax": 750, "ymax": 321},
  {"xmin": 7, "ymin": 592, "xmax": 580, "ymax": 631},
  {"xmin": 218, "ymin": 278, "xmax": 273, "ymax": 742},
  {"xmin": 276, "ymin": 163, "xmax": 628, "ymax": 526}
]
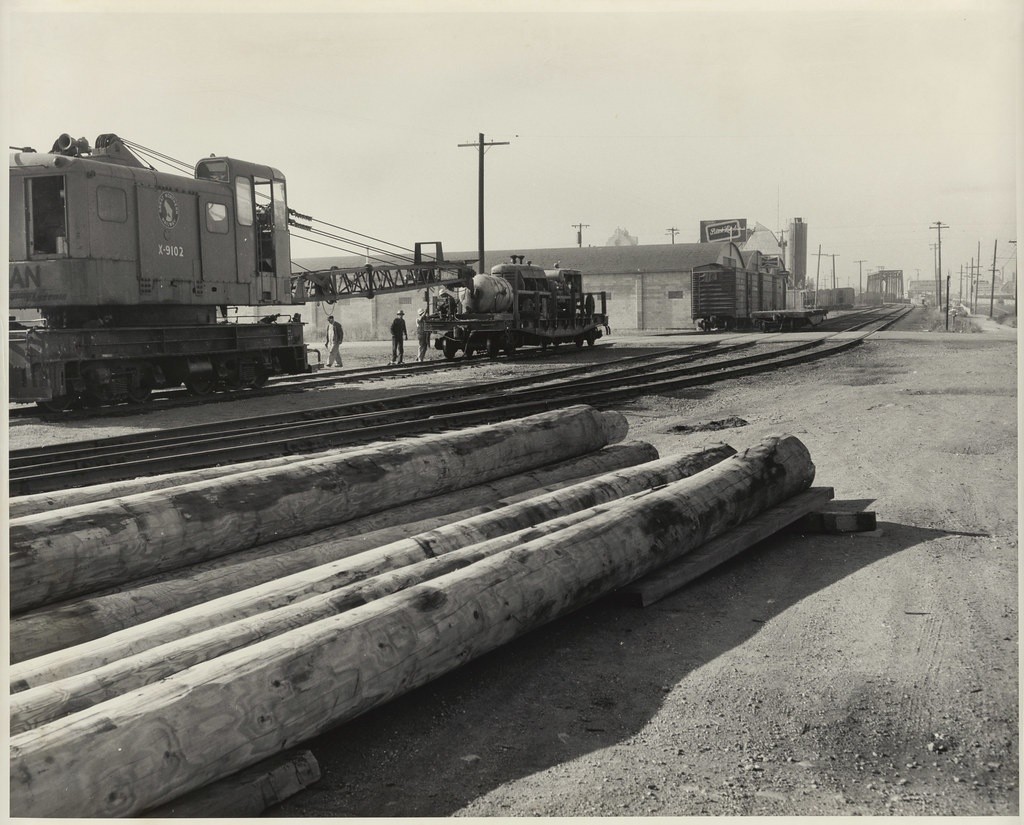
[
  {"xmin": 325, "ymin": 363, "xmax": 332, "ymax": 367},
  {"xmin": 334, "ymin": 364, "xmax": 343, "ymax": 367}
]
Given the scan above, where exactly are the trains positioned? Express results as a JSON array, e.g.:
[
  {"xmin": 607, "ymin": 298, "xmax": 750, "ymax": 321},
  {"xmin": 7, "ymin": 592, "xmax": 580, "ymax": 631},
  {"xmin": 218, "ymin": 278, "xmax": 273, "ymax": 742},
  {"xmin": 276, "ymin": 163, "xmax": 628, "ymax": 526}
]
[
  {"xmin": 691, "ymin": 261, "xmax": 828, "ymax": 333},
  {"xmin": 9, "ymin": 131, "xmax": 611, "ymax": 413}
]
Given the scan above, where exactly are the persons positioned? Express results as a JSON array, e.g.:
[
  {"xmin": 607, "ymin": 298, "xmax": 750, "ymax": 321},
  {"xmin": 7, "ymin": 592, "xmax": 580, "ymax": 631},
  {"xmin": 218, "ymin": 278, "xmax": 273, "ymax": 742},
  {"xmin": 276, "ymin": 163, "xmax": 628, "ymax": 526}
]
[
  {"xmin": 415, "ymin": 308, "xmax": 431, "ymax": 362},
  {"xmin": 390, "ymin": 309, "xmax": 408, "ymax": 362},
  {"xmin": 324, "ymin": 316, "xmax": 343, "ymax": 367}
]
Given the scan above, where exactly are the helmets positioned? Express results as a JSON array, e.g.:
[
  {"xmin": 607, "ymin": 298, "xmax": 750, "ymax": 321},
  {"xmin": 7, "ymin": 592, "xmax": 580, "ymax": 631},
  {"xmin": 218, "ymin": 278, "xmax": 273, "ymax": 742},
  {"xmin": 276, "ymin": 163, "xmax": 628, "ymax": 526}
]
[
  {"xmin": 397, "ymin": 309, "xmax": 405, "ymax": 315},
  {"xmin": 418, "ymin": 308, "xmax": 425, "ymax": 315}
]
